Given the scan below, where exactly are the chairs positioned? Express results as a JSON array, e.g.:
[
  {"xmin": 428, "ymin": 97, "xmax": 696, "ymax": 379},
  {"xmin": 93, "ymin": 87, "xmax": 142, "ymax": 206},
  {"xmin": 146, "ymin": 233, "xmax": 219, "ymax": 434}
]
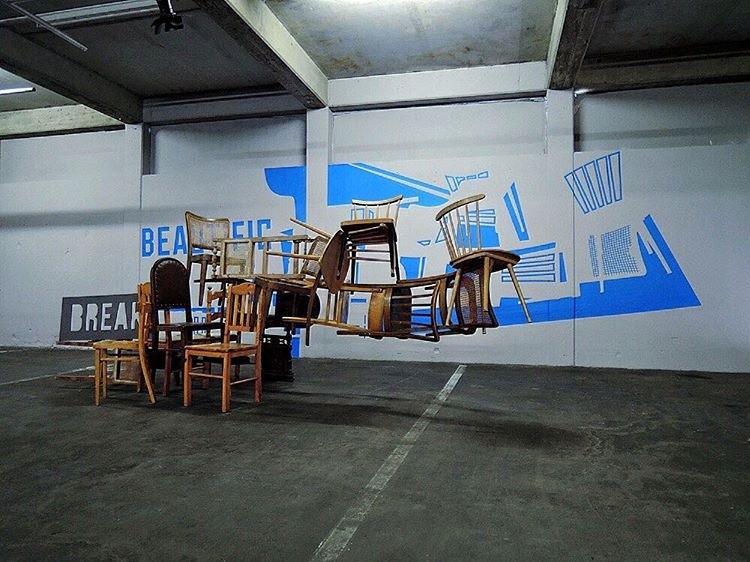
[{"xmin": 95, "ymin": 195, "xmax": 533, "ymax": 412}]
